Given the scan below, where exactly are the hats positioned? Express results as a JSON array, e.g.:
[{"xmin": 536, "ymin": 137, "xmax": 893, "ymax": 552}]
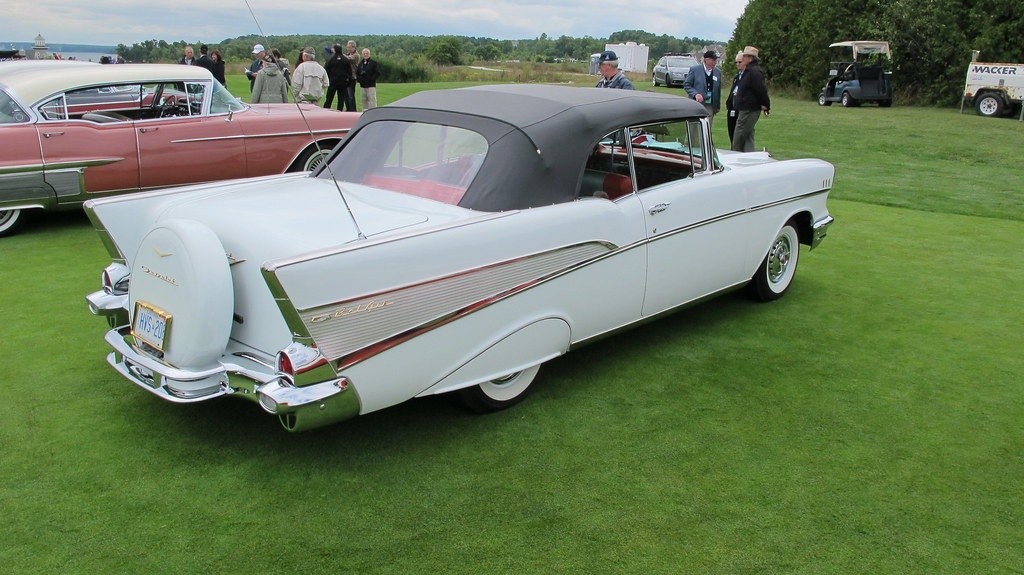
[
  {"xmin": 704, "ymin": 51, "xmax": 721, "ymax": 58},
  {"xmin": 595, "ymin": 51, "xmax": 618, "ymax": 64},
  {"xmin": 739, "ymin": 46, "xmax": 762, "ymax": 61},
  {"xmin": 252, "ymin": 44, "xmax": 264, "ymax": 54}
]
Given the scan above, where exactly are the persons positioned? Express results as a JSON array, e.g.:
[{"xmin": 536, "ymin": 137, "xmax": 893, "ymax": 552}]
[
  {"xmin": 243, "ymin": 40, "xmax": 380, "ymax": 111},
  {"xmin": 726, "ymin": 46, "xmax": 770, "ymax": 152},
  {"xmin": 594, "ymin": 143, "xmax": 605, "ymax": 154},
  {"xmin": 595, "ymin": 51, "xmax": 636, "ymax": 143},
  {"xmin": 52, "ymin": 52, "xmax": 126, "ymax": 65},
  {"xmin": 174, "ymin": 45, "xmax": 225, "ymax": 93},
  {"xmin": 684, "ymin": 51, "xmax": 722, "ymax": 147}
]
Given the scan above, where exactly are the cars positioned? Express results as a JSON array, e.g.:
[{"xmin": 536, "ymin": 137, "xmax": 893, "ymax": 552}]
[
  {"xmin": 40, "ymin": 85, "xmax": 204, "ymax": 113},
  {"xmin": 85, "ymin": 82, "xmax": 836, "ymax": 433},
  {"xmin": 0, "ymin": 59, "xmax": 363, "ymax": 236},
  {"xmin": 652, "ymin": 54, "xmax": 700, "ymax": 87}
]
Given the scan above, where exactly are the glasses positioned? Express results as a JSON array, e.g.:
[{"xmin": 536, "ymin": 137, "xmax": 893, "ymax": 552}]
[{"xmin": 735, "ymin": 61, "xmax": 743, "ymax": 64}]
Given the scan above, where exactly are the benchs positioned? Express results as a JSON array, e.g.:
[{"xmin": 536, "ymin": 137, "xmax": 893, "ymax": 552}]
[
  {"xmin": 457, "ymin": 154, "xmax": 632, "ymax": 199},
  {"xmin": 83, "ymin": 110, "xmax": 135, "ymax": 124}
]
